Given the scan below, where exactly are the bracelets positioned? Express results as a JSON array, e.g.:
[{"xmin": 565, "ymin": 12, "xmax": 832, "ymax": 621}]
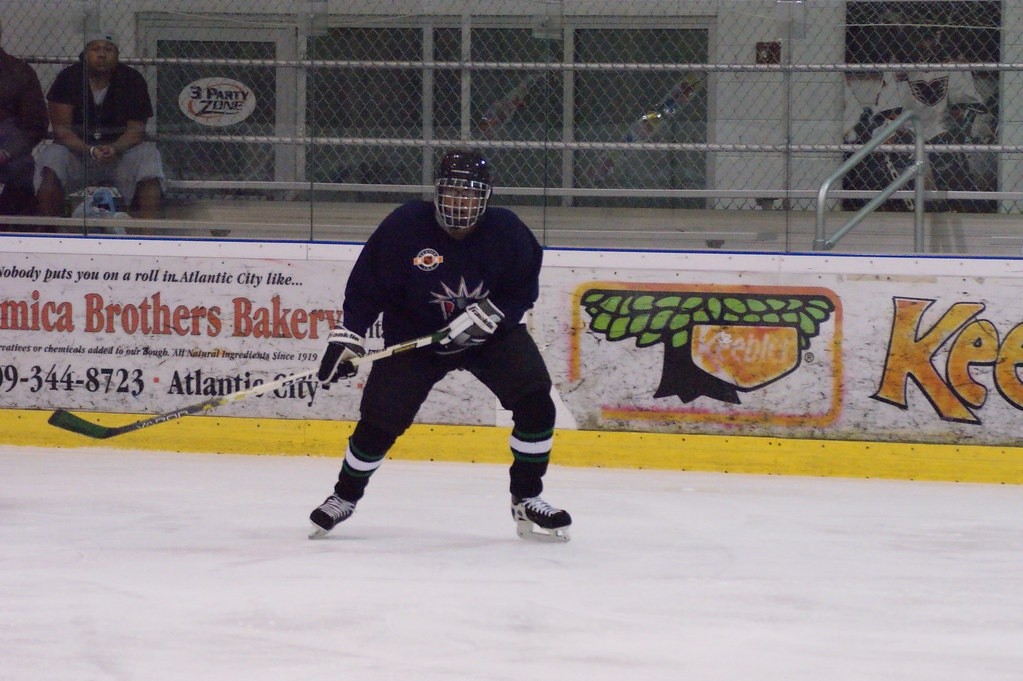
[{"xmin": 90, "ymin": 146, "xmax": 95, "ymax": 159}]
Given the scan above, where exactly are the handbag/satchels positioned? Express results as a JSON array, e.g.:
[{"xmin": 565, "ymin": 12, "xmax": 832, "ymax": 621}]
[{"xmin": 70, "ymin": 189, "xmax": 134, "ymax": 235}]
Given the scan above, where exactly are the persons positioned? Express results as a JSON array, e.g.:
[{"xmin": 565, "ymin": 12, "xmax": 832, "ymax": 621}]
[
  {"xmin": 0, "ymin": 46, "xmax": 49, "ymax": 233},
  {"xmin": 34, "ymin": 32, "xmax": 166, "ymax": 235},
  {"xmin": 843, "ymin": 11, "xmax": 1001, "ymax": 212},
  {"xmin": 309, "ymin": 150, "xmax": 573, "ymax": 543}
]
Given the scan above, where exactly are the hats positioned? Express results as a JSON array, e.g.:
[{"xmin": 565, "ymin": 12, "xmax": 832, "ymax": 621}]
[{"xmin": 79, "ymin": 34, "xmax": 119, "ymax": 60}]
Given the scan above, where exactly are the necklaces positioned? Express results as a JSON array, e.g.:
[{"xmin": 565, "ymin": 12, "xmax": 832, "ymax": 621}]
[{"xmin": 94, "ymin": 87, "xmax": 105, "ymax": 140}]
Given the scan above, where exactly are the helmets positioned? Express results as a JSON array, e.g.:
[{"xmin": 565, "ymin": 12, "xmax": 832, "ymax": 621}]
[{"xmin": 434, "ymin": 150, "xmax": 493, "ymax": 238}]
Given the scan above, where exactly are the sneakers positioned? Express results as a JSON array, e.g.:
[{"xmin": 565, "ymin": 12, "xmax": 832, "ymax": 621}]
[
  {"xmin": 513, "ymin": 496, "xmax": 573, "ymax": 544},
  {"xmin": 308, "ymin": 490, "xmax": 356, "ymax": 542}
]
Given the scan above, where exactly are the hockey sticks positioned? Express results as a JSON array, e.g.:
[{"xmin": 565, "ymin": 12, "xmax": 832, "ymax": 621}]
[{"xmin": 46, "ymin": 331, "xmax": 448, "ymax": 441}]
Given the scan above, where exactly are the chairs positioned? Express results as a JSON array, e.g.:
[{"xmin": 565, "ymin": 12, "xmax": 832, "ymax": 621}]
[{"xmin": 62, "ymin": 185, "xmax": 123, "ymax": 233}]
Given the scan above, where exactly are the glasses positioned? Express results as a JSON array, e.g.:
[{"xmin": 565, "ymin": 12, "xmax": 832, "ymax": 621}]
[{"xmin": 445, "ymin": 189, "xmax": 478, "ymax": 197}]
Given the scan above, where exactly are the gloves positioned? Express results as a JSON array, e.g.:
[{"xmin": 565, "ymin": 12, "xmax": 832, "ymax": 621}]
[
  {"xmin": 318, "ymin": 324, "xmax": 372, "ymax": 390},
  {"xmin": 437, "ymin": 298, "xmax": 506, "ymax": 359}
]
